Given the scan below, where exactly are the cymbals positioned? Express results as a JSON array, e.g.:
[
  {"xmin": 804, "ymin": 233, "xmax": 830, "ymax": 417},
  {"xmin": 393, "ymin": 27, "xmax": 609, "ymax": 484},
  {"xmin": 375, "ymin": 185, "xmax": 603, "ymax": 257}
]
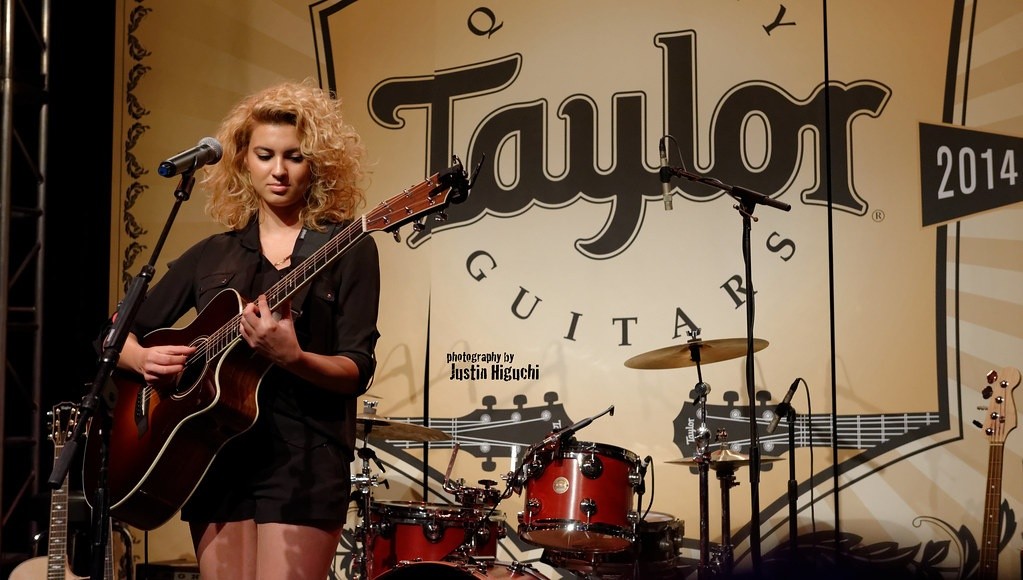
[
  {"xmin": 662, "ymin": 448, "xmax": 788, "ymax": 467},
  {"xmin": 355, "ymin": 411, "xmax": 453, "ymax": 445},
  {"xmin": 623, "ymin": 337, "xmax": 770, "ymax": 371}
]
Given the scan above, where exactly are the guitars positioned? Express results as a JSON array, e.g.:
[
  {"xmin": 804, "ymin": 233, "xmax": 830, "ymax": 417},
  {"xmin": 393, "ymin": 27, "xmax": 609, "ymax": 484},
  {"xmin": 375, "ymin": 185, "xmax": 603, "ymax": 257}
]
[
  {"xmin": 972, "ymin": 366, "xmax": 1022, "ymax": 580},
  {"xmin": 7, "ymin": 400, "xmax": 92, "ymax": 580},
  {"xmin": 103, "ymin": 516, "xmax": 115, "ymax": 580},
  {"xmin": 81, "ymin": 152, "xmax": 486, "ymax": 532}
]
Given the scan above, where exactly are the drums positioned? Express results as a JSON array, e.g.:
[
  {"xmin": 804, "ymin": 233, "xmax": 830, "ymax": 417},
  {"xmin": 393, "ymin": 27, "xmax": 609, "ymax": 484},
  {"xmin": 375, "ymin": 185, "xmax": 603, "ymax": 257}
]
[
  {"xmin": 370, "ymin": 556, "xmax": 553, "ymax": 580},
  {"xmin": 516, "ymin": 439, "xmax": 641, "ymax": 555},
  {"xmin": 539, "ymin": 508, "xmax": 685, "ymax": 579},
  {"xmin": 364, "ymin": 498, "xmax": 507, "ymax": 580}
]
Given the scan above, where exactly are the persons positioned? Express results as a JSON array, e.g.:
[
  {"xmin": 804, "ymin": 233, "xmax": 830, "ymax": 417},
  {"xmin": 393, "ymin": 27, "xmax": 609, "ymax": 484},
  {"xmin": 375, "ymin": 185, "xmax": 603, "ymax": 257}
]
[{"xmin": 94, "ymin": 72, "xmax": 380, "ymax": 580}]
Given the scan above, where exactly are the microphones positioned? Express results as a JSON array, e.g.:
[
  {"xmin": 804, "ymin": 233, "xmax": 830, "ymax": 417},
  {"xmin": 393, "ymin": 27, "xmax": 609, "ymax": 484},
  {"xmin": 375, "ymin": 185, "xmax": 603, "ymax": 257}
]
[
  {"xmin": 766, "ymin": 378, "xmax": 800, "ymax": 434},
  {"xmin": 633, "ymin": 457, "xmax": 652, "ymax": 493},
  {"xmin": 610, "ymin": 404, "xmax": 614, "ymax": 415},
  {"xmin": 659, "ymin": 138, "xmax": 673, "ymax": 211},
  {"xmin": 158, "ymin": 137, "xmax": 223, "ymax": 178}
]
